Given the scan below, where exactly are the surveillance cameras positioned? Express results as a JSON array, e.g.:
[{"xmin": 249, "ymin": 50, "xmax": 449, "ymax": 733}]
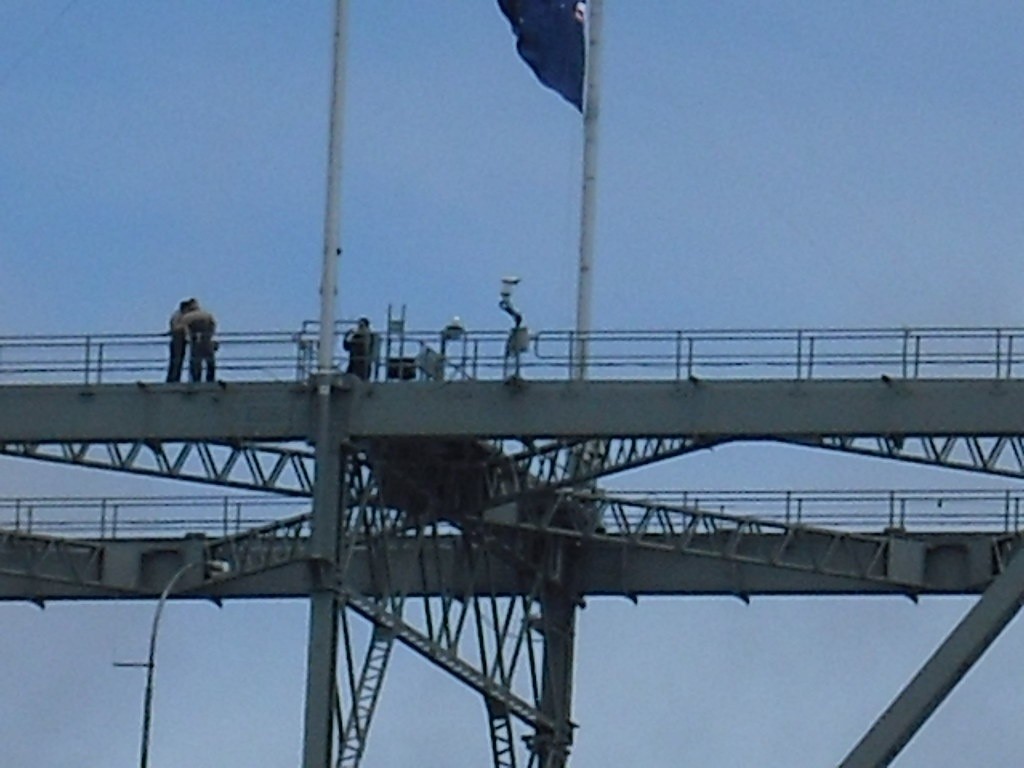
[{"xmin": 502, "ymin": 276, "xmax": 521, "ymax": 285}]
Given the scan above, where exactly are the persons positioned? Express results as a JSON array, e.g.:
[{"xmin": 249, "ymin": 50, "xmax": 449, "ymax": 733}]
[
  {"xmin": 165, "ymin": 299, "xmax": 217, "ymax": 384},
  {"xmin": 343, "ymin": 319, "xmax": 376, "ymax": 381}
]
